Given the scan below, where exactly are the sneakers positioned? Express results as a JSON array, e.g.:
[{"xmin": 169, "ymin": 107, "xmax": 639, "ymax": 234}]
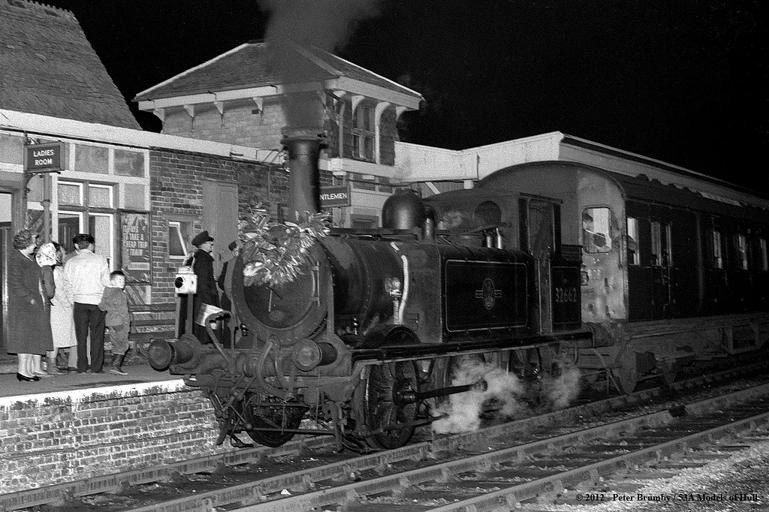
[
  {"xmin": 78, "ymin": 370, "xmax": 109, "ymax": 376},
  {"xmin": 16, "ymin": 369, "xmax": 68, "ymax": 382}
]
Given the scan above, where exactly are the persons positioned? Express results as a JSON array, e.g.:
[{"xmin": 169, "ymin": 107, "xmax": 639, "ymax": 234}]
[
  {"xmin": 217, "ymin": 240, "xmax": 239, "ymax": 349},
  {"xmin": 592, "ymin": 231, "xmax": 610, "ymax": 251},
  {"xmin": 97, "ymin": 269, "xmax": 132, "ymax": 377},
  {"xmin": 582, "ymin": 212, "xmax": 598, "ymax": 253},
  {"xmin": 64, "ymin": 236, "xmax": 81, "ymax": 266},
  {"xmin": 35, "ymin": 241, "xmax": 57, "ymax": 363},
  {"xmin": 48, "ymin": 241, "xmax": 80, "ymax": 373},
  {"xmin": 179, "ymin": 229, "xmax": 220, "ymax": 344},
  {"xmin": 5, "ymin": 229, "xmax": 53, "ymax": 383},
  {"xmin": 65, "ymin": 233, "xmax": 114, "ymax": 374}
]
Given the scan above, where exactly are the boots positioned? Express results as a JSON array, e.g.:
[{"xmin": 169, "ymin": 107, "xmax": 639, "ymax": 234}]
[{"xmin": 110, "ymin": 353, "xmax": 128, "ymax": 376}]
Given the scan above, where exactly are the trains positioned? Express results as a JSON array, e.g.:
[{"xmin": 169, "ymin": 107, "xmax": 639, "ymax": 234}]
[{"xmin": 143, "ymin": 131, "xmax": 768, "ymax": 452}]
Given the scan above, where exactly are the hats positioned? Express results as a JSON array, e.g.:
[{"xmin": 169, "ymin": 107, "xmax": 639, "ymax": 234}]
[{"xmin": 191, "ymin": 231, "xmax": 213, "ymax": 245}]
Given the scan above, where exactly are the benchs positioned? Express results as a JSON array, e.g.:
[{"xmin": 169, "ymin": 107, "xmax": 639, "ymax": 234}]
[{"xmin": 103, "ymin": 302, "xmax": 176, "ymax": 366}]
